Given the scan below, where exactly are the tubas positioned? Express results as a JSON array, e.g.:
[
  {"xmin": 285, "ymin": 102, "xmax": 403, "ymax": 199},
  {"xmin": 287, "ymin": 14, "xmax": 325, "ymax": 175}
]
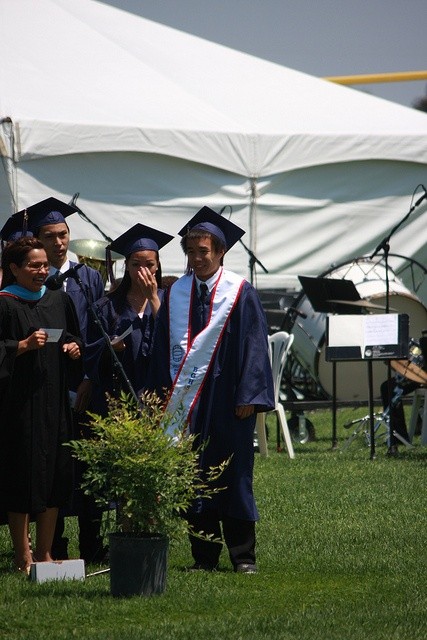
[{"xmin": 69, "ymin": 235, "xmax": 127, "ymax": 294}]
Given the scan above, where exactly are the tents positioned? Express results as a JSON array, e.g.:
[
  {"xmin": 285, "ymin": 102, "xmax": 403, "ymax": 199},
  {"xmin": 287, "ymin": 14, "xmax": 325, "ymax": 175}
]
[{"xmin": 0, "ymin": 2, "xmax": 427, "ymax": 313}]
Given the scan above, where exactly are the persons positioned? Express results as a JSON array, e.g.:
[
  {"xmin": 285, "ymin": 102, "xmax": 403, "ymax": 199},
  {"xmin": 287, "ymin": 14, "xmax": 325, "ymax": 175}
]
[
  {"xmin": 154, "ymin": 205, "xmax": 275, "ymax": 576},
  {"xmin": 88, "ymin": 224, "xmax": 172, "ymax": 525},
  {"xmin": 0, "ymin": 195, "xmax": 107, "ymax": 577}
]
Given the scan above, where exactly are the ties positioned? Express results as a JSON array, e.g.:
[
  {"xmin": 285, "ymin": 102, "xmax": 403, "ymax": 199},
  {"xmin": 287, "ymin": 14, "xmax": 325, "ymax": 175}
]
[{"xmin": 200, "ymin": 283, "xmax": 208, "ymax": 304}]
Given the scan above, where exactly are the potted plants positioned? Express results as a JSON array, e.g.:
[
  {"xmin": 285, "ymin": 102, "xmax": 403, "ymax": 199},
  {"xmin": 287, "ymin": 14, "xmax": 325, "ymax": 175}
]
[{"xmin": 67, "ymin": 390, "xmax": 233, "ymax": 591}]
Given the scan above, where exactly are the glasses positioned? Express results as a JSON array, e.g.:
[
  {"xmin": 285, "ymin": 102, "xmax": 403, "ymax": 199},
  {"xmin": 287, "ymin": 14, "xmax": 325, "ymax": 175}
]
[{"xmin": 22, "ymin": 261, "xmax": 50, "ymax": 269}]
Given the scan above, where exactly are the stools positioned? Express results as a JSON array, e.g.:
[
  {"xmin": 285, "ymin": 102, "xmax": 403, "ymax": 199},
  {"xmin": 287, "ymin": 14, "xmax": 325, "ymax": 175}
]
[{"xmin": 408, "ymin": 387, "xmax": 427, "ymax": 446}]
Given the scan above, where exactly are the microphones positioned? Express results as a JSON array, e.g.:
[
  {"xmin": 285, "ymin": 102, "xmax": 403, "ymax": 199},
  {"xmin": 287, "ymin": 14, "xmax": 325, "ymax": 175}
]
[{"xmin": 46, "ymin": 261, "xmax": 85, "ymax": 292}]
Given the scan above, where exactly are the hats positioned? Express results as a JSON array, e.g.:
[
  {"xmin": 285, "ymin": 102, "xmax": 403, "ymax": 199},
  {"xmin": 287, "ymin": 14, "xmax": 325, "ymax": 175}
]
[
  {"xmin": 178, "ymin": 206, "xmax": 246, "ymax": 276},
  {"xmin": 0, "ymin": 196, "xmax": 78, "ymax": 241},
  {"xmin": 105, "ymin": 223, "xmax": 175, "ymax": 288}
]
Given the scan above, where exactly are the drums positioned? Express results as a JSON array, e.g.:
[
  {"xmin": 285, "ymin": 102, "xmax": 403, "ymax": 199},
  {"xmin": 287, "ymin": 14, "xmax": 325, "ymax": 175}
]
[{"xmin": 390, "ymin": 338, "xmax": 427, "ymax": 384}]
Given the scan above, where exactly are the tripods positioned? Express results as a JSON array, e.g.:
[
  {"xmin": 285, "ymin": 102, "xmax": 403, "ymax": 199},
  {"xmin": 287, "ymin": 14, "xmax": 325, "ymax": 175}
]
[{"xmin": 347, "ymin": 361, "xmax": 427, "ymax": 455}]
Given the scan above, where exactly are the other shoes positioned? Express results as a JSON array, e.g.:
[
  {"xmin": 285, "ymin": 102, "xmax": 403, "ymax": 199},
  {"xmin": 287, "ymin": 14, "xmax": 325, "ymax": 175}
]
[
  {"xmin": 192, "ymin": 563, "xmax": 215, "ymax": 570},
  {"xmin": 237, "ymin": 563, "xmax": 257, "ymax": 572},
  {"xmin": 14, "ymin": 556, "xmax": 32, "ymax": 575}
]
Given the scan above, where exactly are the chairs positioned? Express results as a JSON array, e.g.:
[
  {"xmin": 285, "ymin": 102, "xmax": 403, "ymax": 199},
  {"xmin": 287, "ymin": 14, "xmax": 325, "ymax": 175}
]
[{"xmin": 255, "ymin": 331, "xmax": 298, "ymax": 459}]
